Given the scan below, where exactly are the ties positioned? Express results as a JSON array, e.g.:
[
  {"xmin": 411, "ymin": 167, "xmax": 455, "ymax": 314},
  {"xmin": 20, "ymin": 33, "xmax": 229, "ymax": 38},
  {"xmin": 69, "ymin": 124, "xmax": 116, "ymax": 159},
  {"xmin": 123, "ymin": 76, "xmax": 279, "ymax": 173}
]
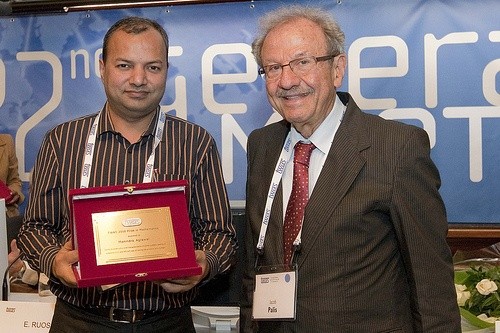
[{"xmin": 285, "ymin": 142, "xmax": 317, "ymax": 271}]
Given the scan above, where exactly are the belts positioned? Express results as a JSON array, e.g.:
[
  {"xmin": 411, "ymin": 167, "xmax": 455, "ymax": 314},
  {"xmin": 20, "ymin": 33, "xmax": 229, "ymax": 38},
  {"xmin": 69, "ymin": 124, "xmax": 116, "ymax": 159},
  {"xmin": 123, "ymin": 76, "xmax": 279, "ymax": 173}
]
[{"xmin": 85, "ymin": 307, "xmax": 183, "ymax": 324}]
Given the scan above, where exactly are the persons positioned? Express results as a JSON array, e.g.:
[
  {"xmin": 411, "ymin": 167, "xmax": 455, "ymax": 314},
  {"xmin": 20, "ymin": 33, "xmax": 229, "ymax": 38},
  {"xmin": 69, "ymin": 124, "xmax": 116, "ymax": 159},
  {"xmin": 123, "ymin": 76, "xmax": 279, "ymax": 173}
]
[
  {"xmin": 211, "ymin": 11, "xmax": 463, "ymax": 333},
  {"xmin": 15, "ymin": 17, "xmax": 239, "ymax": 333},
  {"xmin": 0, "ymin": 134, "xmax": 51, "ymax": 293}
]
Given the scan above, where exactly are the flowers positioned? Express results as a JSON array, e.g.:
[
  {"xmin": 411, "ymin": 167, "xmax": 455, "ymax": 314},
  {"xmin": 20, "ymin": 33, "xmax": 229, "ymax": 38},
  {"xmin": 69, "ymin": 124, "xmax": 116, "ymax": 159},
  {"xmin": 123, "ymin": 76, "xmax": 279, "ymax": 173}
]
[{"xmin": 454, "ymin": 262, "xmax": 500, "ymax": 324}]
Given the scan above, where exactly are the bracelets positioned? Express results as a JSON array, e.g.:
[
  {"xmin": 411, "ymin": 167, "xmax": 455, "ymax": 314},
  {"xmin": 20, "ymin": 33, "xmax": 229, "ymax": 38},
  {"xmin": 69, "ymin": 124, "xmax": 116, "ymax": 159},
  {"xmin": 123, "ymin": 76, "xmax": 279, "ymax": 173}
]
[{"xmin": 13, "ymin": 262, "xmax": 26, "ymax": 278}]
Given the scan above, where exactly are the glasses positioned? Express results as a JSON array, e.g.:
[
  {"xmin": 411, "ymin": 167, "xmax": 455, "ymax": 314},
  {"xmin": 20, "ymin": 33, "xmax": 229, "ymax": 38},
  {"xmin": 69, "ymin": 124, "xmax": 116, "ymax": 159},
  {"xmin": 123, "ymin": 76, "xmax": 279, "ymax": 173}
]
[{"xmin": 257, "ymin": 55, "xmax": 334, "ymax": 81}]
